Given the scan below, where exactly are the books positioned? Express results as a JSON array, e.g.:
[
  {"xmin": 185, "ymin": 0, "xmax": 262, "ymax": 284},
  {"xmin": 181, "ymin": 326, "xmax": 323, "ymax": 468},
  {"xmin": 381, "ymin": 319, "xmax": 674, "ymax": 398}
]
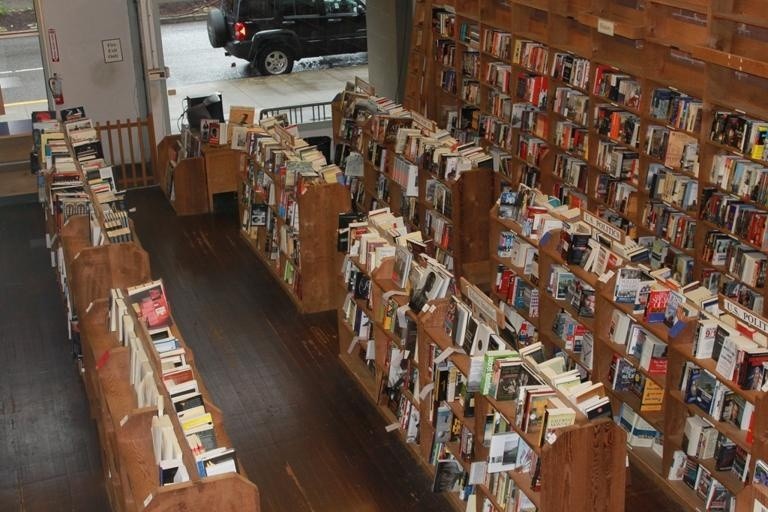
[
  {"xmin": 107, "ymin": 279, "xmax": 236, "ymax": 487},
  {"xmin": 337, "ymin": 206, "xmax": 614, "ymax": 512},
  {"xmin": 490, "ymin": 183, "xmax": 765, "ymax": 512},
  {"xmin": 162, "ymin": 116, "xmax": 230, "ymax": 204},
  {"xmin": 331, "ymin": 75, "xmax": 495, "ymax": 272},
  {"xmin": 228, "ymin": 105, "xmax": 345, "ymax": 300},
  {"xmin": 45, "ymin": 232, "xmax": 85, "ymax": 361},
  {"xmin": 31, "ymin": 106, "xmax": 136, "ymax": 246},
  {"xmin": 430, "ymin": 7, "xmax": 557, "ymax": 188},
  {"xmin": 551, "ymin": 51, "xmax": 765, "ymax": 317},
  {"xmin": 236, "ymin": 1, "xmax": 765, "ymax": 512}
]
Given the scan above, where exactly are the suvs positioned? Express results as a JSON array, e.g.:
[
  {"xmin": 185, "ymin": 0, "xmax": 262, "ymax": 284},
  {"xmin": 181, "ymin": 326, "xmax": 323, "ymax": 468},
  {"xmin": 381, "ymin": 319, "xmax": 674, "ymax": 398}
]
[{"xmin": 205, "ymin": 0, "xmax": 367, "ymax": 79}]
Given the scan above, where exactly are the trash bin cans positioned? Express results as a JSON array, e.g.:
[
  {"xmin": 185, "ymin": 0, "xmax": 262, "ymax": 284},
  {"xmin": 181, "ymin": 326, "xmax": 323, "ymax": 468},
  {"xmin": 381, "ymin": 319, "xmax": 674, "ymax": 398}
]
[{"xmin": 303, "ymin": 136, "xmax": 331, "ymax": 165}]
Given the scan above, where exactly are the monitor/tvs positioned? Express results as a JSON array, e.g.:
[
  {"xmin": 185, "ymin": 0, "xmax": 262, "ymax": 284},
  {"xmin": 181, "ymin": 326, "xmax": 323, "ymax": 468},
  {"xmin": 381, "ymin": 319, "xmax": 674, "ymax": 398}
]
[{"xmin": 187, "ymin": 94, "xmax": 224, "ymax": 130}]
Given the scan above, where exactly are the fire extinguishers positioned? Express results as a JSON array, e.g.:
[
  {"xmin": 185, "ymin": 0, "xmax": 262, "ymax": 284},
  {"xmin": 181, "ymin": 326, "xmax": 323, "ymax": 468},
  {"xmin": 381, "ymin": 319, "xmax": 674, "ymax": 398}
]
[{"xmin": 48, "ymin": 72, "xmax": 64, "ymax": 105}]
